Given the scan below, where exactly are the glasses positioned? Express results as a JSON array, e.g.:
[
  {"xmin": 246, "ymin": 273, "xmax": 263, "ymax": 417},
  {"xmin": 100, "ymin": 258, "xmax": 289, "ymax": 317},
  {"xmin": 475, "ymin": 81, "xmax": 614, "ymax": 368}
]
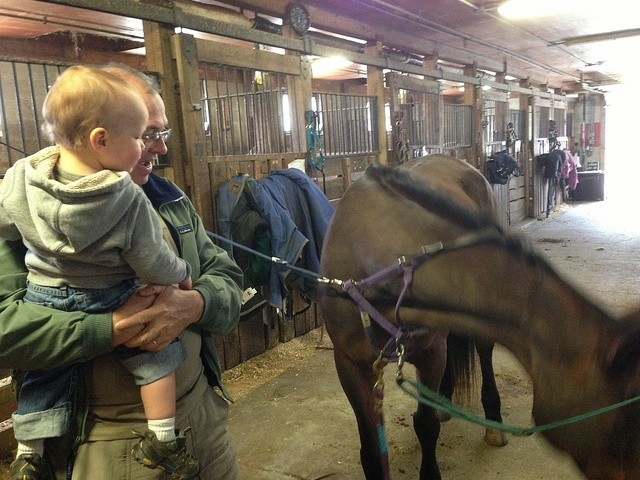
[{"xmin": 142, "ymin": 127, "xmax": 172, "ymax": 148}]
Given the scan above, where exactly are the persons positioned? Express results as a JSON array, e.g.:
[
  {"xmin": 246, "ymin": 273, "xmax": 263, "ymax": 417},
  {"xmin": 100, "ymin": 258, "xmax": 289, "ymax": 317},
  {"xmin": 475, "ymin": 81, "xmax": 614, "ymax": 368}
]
[
  {"xmin": 1, "ymin": 64, "xmax": 194, "ymax": 480},
  {"xmin": 0, "ymin": 65, "xmax": 245, "ymax": 480}
]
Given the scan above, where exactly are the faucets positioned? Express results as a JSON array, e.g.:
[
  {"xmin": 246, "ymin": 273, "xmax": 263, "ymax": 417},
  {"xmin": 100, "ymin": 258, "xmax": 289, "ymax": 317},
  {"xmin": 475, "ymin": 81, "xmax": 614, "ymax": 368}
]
[{"xmin": 585, "ymin": 145, "xmax": 593, "ymax": 157}]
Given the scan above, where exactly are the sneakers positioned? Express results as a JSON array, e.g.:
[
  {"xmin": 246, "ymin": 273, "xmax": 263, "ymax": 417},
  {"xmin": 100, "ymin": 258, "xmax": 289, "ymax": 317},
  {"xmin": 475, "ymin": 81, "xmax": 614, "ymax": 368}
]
[
  {"xmin": 9, "ymin": 448, "xmax": 47, "ymax": 480},
  {"xmin": 130, "ymin": 426, "xmax": 199, "ymax": 480}
]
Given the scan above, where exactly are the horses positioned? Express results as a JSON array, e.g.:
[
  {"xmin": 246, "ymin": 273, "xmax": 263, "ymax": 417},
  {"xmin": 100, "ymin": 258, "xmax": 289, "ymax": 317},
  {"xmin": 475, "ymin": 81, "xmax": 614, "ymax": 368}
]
[{"xmin": 317, "ymin": 153, "xmax": 640, "ymax": 480}]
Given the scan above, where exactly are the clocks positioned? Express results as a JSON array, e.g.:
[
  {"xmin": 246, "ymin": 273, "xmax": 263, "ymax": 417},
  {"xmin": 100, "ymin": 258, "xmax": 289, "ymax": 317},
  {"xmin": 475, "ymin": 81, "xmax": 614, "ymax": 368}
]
[{"xmin": 286, "ymin": 2, "xmax": 311, "ymax": 34}]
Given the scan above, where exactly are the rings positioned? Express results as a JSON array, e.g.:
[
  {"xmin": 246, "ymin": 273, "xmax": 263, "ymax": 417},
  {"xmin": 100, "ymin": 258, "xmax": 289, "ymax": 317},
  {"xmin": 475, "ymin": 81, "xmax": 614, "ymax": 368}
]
[{"xmin": 152, "ymin": 339, "xmax": 158, "ymax": 346}]
